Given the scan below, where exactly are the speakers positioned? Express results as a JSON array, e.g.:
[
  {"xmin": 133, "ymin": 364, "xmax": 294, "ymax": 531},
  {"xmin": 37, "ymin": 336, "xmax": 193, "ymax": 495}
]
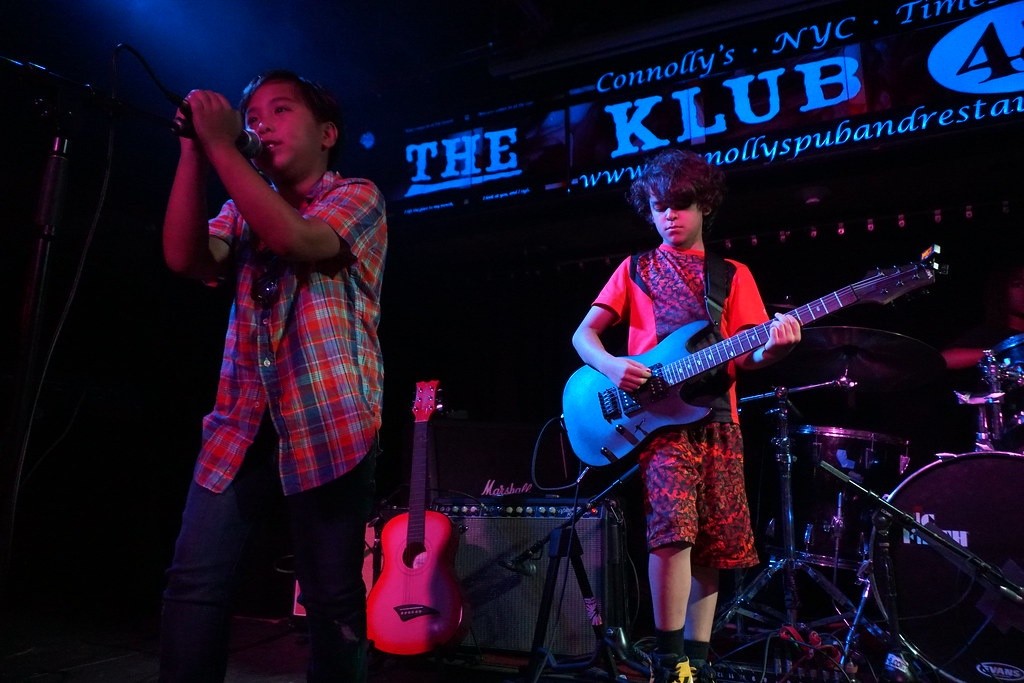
[{"xmin": 379, "ymin": 496, "xmax": 627, "ymax": 665}]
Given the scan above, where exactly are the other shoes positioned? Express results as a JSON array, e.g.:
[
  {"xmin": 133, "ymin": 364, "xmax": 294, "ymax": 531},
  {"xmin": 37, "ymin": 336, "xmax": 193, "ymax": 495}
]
[{"xmin": 652, "ymin": 652, "xmax": 716, "ymax": 683}]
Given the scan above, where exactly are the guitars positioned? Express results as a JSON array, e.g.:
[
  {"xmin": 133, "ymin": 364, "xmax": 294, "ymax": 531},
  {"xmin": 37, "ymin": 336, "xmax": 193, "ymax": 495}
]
[
  {"xmin": 363, "ymin": 377, "xmax": 469, "ymax": 655},
  {"xmin": 560, "ymin": 241, "xmax": 945, "ymax": 467}
]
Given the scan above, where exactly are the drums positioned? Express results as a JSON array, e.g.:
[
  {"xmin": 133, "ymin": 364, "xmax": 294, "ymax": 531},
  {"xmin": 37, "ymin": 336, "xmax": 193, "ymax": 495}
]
[
  {"xmin": 977, "ymin": 332, "xmax": 1024, "ymax": 447},
  {"xmin": 866, "ymin": 448, "xmax": 1024, "ymax": 683},
  {"xmin": 783, "ymin": 422, "xmax": 916, "ymax": 575}
]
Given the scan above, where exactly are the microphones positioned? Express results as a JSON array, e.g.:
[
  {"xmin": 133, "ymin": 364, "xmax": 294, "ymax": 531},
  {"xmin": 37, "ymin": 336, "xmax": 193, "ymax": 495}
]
[
  {"xmin": 498, "ymin": 559, "xmax": 536, "ymax": 576},
  {"xmin": 178, "ymin": 100, "xmax": 264, "ymax": 159}
]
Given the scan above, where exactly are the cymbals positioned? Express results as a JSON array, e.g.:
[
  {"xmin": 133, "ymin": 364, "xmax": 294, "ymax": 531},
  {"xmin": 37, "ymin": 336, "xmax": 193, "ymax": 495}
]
[{"xmin": 799, "ymin": 322, "xmax": 949, "ymax": 387}]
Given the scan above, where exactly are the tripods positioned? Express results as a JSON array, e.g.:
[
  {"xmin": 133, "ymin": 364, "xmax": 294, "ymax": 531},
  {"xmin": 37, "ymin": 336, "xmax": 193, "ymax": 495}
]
[
  {"xmin": 512, "ymin": 463, "xmax": 670, "ymax": 683},
  {"xmin": 706, "ymin": 363, "xmax": 1024, "ymax": 683}
]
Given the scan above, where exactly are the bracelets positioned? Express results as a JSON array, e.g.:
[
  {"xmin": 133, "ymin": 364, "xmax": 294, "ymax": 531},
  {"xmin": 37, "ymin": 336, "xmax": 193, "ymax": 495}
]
[{"xmin": 753, "ymin": 347, "xmax": 766, "ymax": 368}]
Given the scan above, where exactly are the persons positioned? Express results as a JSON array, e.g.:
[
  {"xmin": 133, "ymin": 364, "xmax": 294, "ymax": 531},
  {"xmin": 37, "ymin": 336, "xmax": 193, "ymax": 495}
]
[
  {"xmin": 161, "ymin": 71, "xmax": 390, "ymax": 681},
  {"xmin": 570, "ymin": 150, "xmax": 802, "ymax": 683},
  {"xmin": 910, "ymin": 267, "xmax": 1024, "ymax": 395}
]
